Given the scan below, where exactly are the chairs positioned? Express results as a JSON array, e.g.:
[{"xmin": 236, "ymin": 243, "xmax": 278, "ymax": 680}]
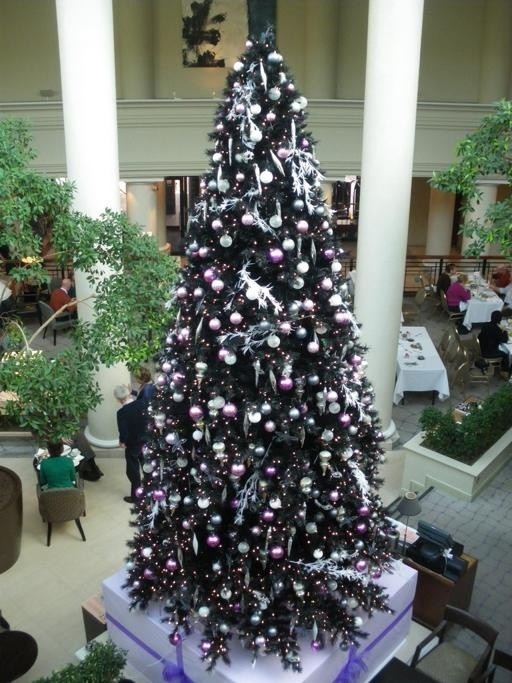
[
  {"xmin": 36, "ymin": 478, "xmax": 87, "ymax": 545},
  {"xmin": 38, "ymin": 276, "xmax": 78, "ymax": 345},
  {"xmin": 410, "ymin": 604, "xmax": 511, "ymax": 682},
  {"xmin": 402, "ymin": 274, "xmax": 502, "ymax": 400}
]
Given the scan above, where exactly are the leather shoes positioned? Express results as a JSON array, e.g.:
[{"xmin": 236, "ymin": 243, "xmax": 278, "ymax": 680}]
[{"xmin": 124, "ymin": 496, "xmax": 137, "ymax": 504}]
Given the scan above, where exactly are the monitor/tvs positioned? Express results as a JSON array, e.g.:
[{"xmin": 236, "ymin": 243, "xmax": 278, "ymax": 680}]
[{"xmin": 416, "ymin": 520, "xmax": 456, "ymax": 549}]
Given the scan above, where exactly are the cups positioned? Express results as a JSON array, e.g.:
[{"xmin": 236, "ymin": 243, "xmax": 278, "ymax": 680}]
[{"xmin": 72, "ymin": 449, "xmax": 78, "ymax": 455}]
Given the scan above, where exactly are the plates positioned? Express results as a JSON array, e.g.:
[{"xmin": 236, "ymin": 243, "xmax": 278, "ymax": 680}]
[{"xmin": 69, "ymin": 452, "xmax": 84, "ymax": 467}]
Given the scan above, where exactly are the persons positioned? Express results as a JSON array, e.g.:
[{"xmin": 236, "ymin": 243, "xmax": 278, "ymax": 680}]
[
  {"xmin": 114, "ymin": 384, "xmax": 150, "ymax": 503},
  {"xmin": 434, "ymin": 264, "xmax": 512, "ymax": 381},
  {"xmin": 36, "ymin": 437, "xmax": 77, "ymax": 492},
  {"xmin": 49, "ymin": 279, "xmax": 78, "ymax": 323},
  {"xmin": 121, "ymin": 367, "xmax": 158, "ymax": 410}
]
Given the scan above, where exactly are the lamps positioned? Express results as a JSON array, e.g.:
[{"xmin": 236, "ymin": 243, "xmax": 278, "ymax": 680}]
[{"xmin": 396, "ymin": 492, "xmax": 422, "ymax": 556}]
